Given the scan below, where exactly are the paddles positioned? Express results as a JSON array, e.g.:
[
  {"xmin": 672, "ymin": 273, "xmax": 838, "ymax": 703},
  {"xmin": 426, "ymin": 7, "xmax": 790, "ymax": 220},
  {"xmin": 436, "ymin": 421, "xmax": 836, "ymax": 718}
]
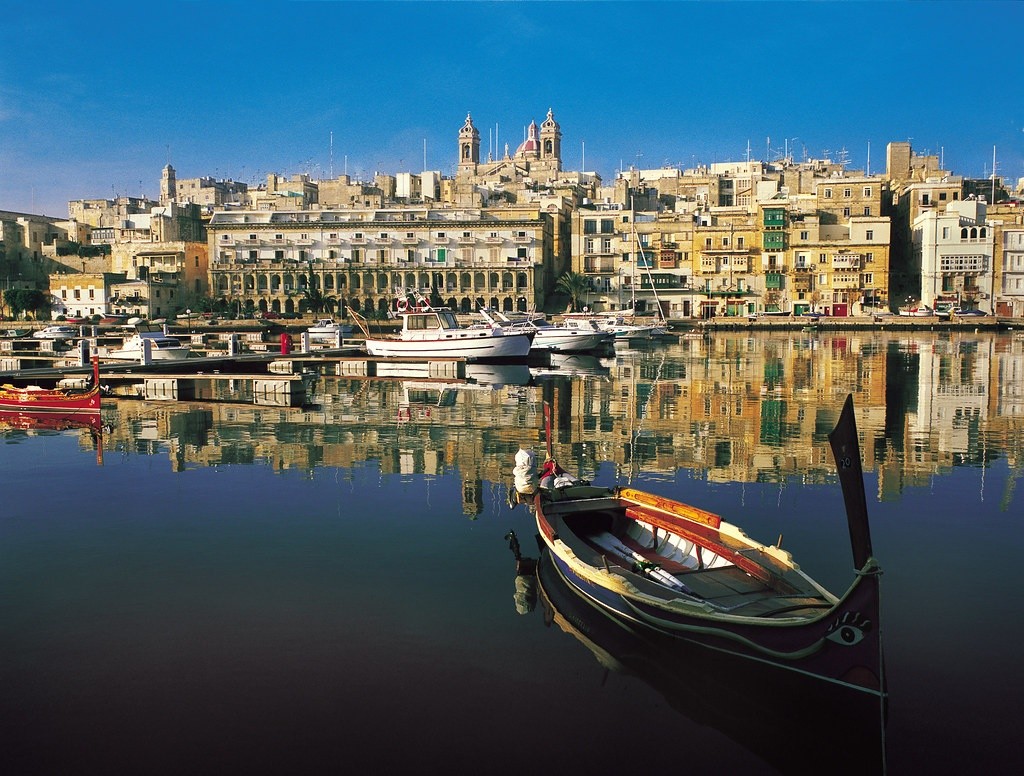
[{"xmin": 585, "ymin": 530, "xmax": 705, "ymax": 599}]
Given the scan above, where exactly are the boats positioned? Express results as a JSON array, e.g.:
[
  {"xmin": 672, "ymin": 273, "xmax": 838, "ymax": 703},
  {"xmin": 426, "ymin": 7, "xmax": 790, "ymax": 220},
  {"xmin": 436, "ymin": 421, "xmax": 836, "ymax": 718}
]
[
  {"xmin": 503, "ymin": 528, "xmax": 889, "ymax": 776},
  {"xmin": 748, "ymin": 308, "xmax": 988, "ymax": 319},
  {"xmin": 0, "ymin": 410, "xmax": 104, "ymax": 467},
  {"xmin": 0, "ymin": 354, "xmax": 101, "ymax": 410},
  {"xmin": 1, "ymin": 314, "xmax": 90, "ymax": 338},
  {"xmin": 510, "ymin": 393, "xmax": 889, "ymax": 702},
  {"xmin": 107, "ymin": 287, "xmax": 671, "ymax": 362}
]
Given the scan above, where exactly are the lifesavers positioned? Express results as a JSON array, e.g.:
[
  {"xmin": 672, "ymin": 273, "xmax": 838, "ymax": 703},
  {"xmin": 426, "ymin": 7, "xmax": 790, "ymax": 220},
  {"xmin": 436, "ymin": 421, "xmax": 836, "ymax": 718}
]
[
  {"xmin": 397, "ymin": 297, "xmax": 409, "ymax": 311},
  {"xmin": 417, "ymin": 297, "xmax": 430, "ymax": 312},
  {"xmin": 398, "ymin": 408, "xmax": 410, "ymax": 423}
]
